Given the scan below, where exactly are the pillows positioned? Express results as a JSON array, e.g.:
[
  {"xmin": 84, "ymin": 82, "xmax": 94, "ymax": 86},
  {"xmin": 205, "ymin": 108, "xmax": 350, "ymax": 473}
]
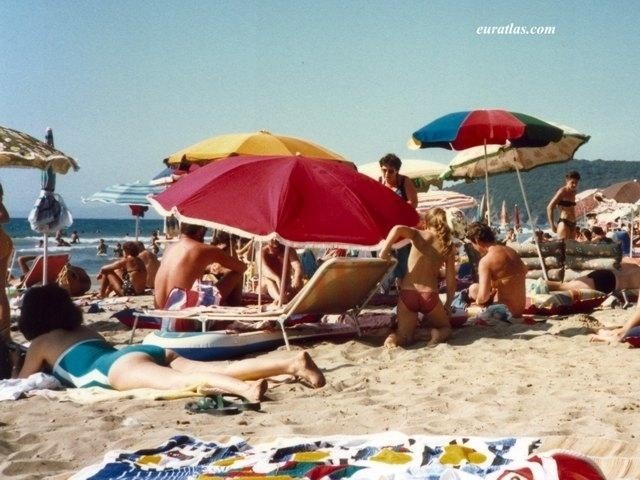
[{"xmin": 538, "ymin": 290, "xmax": 607, "ymax": 314}]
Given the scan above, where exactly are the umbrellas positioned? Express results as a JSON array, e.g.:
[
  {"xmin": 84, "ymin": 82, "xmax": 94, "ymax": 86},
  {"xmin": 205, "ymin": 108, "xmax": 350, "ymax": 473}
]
[
  {"xmin": 82, "ymin": 180, "xmax": 167, "ymax": 240},
  {"xmin": 500, "ymin": 201, "xmax": 511, "ymax": 228},
  {"xmin": 514, "ymin": 206, "xmax": 520, "ymax": 230},
  {"xmin": 148, "ymin": 165, "xmax": 194, "ymax": 186},
  {"xmin": 572, "ymin": 188, "xmax": 601, "ymax": 217},
  {"xmin": 413, "ymin": 191, "xmax": 479, "ymax": 212},
  {"xmin": 478, "ymin": 193, "xmax": 492, "ymax": 223},
  {"xmin": 28, "ymin": 128, "xmax": 73, "ymax": 285},
  {"xmin": 408, "ymin": 110, "xmax": 566, "ymax": 226},
  {"xmin": 437, "ymin": 123, "xmax": 591, "ymax": 279},
  {"xmin": 0, "ymin": 126, "xmax": 81, "ymax": 175},
  {"xmin": 357, "ymin": 156, "xmax": 452, "ymax": 192},
  {"xmin": 146, "ymin": 155, "xmax": 423, "ymax": 303},
  {"xmin": 163, "ymin": 131, "xmax": 351, "ymax": 165},
  {"xmin": 595, "ymin": 179, "xmax": 640, "ymax": 256}
]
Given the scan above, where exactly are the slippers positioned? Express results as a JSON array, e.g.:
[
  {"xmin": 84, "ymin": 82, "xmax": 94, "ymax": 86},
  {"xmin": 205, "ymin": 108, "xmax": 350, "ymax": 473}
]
[
  {"xmin": 184, "ymin": 393, "xmax": 237, "ymax": 416},
  {"xmin": 213, "ymin": 392, "xmax": 261, "ymax": 410}
]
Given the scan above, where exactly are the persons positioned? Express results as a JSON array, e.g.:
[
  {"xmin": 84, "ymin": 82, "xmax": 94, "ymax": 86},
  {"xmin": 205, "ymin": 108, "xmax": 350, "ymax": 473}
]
[
  {"xmin": 466, "ymin": 223, "xmax": 527, "ymax": 319},
  {"xmin": 255, "ymin": 237, "xmax": 301, "ymax": 305},
  {"xmin": 154, "ymin": 221, "xmax": 246, "ymax": 311},
  {"xmin": 574, "ymin": 222, "xmax": 630, "ymax": 254},
  {"xmin": 83, "ymin": 230, "xmax": 84, "ymax": 232},
  {"xmin": 36, "ymin": 239, "xmax": 43, "ymax": 247},
  {"xmin": 383, "ymin": 207, "xmax": 452, "ymax": 350},
  {"xmin": 547, "ymin": 263, "xmax": 640, "ymax": 290},
  {"xmin": 7, "ymin": 254, "xmax": 38, "ymax": 287},
  {"xmin": 94, "ymin": 242, "xmax": 147, "ymax": 299},
  {"xmin": 133, "ymin": 242, "xmax": 159, "ymax": 290},
  {"xmin": 377, "ymin": 155, "xmax": 417, "ymax": 287},
  {"xmin": 55, "ymin": 227, "xmax": 72, "ymax": 245},
  {"xmin": 69, "ymin": 231, "xmax": 79, "ymax": 242},
  {"xmin": 152, "ymin": 233, "xmax": 160, "ymax": 258},
  {"xmin": 9, "ymin": 283, "xmax": 326, "ymax": 405},
  {"xmin": 547, "ymin": 171, "xmax": 580, "ymax": 240},
  {"xmin": 586, "ymin": 290, "xmax": 640, "ymax": 343},
  {"xmin": 0, "ymin": 184, "xmax": 14, "ymax": 351},
  {"xmin": 318, "ymin": 249, "xmax": 358, "ymax": 262},
  {"xmin": 113, "ymin": 242, "xmax": 124, "ymax": 255},
  {"xmin": 97, "ymin": 240, "xmax": 109, "ymax": 256},
  {"xmin": 532, "ymin": 229, "xmax": 553, "ymax": 244},
  {"xmin": 206, "ymin": 228, "xmax": 253, "ymax": 277},
  {"xmin": 502, "ymin": 229, "xmax": 515, "ymax": 243},
  {"xmin": 157, "ymin": 227, "xmax": 160, "ymax": 233}
]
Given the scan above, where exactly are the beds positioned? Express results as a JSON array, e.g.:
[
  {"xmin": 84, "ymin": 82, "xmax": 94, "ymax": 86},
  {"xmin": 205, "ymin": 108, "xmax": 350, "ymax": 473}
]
[
  {"xmin": 16, "ymin": 255, "xmax": 69, "ymax": 294},
  {"xmin": 129, "ymin": 258, "xmax": 398, "ymax": 353}
]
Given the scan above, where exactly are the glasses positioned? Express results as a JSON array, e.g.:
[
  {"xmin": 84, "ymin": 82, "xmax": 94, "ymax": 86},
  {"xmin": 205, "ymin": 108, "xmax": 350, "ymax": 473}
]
[{"xmin": 381, "ymin": 168, "xmax": 398, "ymax": 173}]
